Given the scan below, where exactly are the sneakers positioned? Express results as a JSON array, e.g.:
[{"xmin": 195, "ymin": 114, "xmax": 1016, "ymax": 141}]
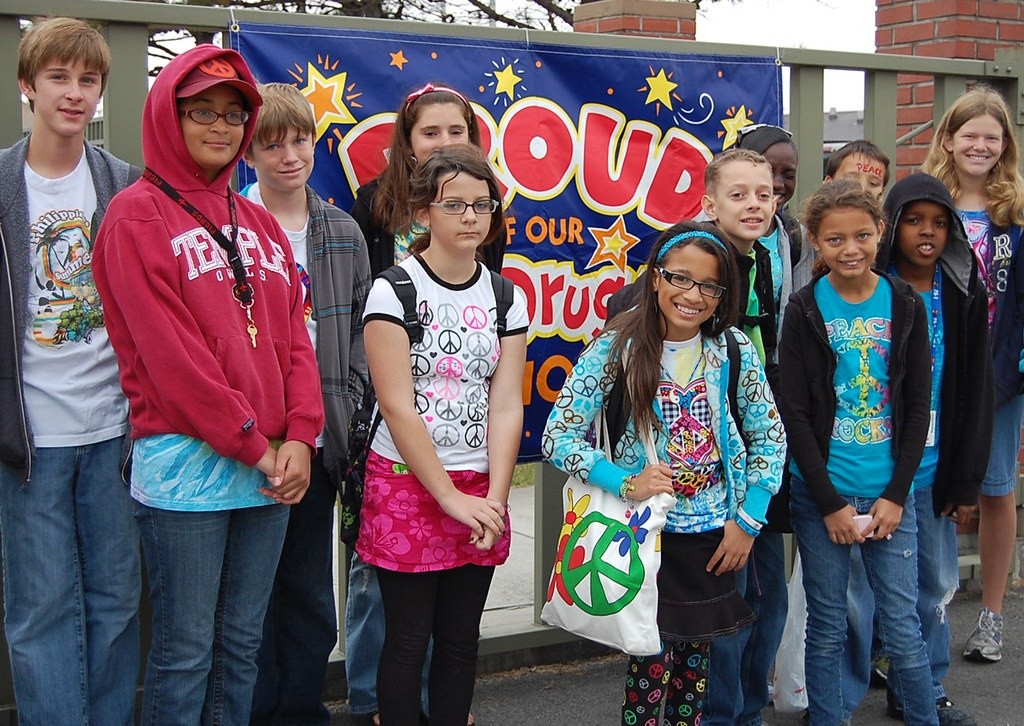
[
  {"xmin": 962, "ymin": 607, "xmax": 1004, "ymax": 663},
  {"xmin": 884, "ymin": 683, "xmax": 976, "ymax": 726}
]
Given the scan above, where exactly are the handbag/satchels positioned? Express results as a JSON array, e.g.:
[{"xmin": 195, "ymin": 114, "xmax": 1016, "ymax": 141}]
[
  {"xmin": 541, "ymin": 345, "xmax": 661, "ymax": 657},
  {"xmin": 770, "ymin": 547, "xmax": 811, "ymax": 711}
]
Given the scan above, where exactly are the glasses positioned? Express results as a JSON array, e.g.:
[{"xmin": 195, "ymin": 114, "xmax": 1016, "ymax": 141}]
[
  {"xmin": 428, "ymin": 198, "xmax": 499, "ymax": 215},
  {"xmin": 654, "ymin": 263, "xmax": 726, "ymax": 299},
  {"xmin": 178, "ymin": 107, "xmax": 253, "ymax": 125}
]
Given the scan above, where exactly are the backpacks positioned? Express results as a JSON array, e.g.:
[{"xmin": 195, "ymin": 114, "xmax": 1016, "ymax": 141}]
[{"xmin": 340, "ymin": 266, "xmax": 511, "ymax": 548}]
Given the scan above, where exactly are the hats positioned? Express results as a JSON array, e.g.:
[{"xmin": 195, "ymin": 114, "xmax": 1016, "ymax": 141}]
[
  {"xmin": 175, "ymin": 57, "xmax": 264, "ymax": 107},
  {"xmin": 740, "ymin": 126, "xmax": 796, "ymax": 156}
]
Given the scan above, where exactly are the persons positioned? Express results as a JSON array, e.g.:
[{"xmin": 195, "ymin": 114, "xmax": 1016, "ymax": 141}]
[
  {"xmin": 356, "ymin": 147, "xmax": 530, "ymax": 726},
  {"xmin": 342, "ymin": 81, "xmax": 507, "ymax": 726},
  {"xmin": 237, "ymin": 82, "xmax": 372, "ymax": 726},
  {"xmin": 542, "ymin": 219, "xmax": 788, "ymax": 726},
  {"xmin": 90, "ymin": 41, "xmax": 324, "ymax": 726},
  {"xmin": 608, "ymin": 92, "xmax": 1024, "ymax": 726},
  {"xmin": 0, "ymin": 15, "xmax": 143, "ymax": 726}
]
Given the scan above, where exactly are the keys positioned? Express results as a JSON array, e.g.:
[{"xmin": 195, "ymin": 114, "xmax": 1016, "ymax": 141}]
[{"xmin": 247, "ymin": 319, "xmax": 257, "ymax": 348}]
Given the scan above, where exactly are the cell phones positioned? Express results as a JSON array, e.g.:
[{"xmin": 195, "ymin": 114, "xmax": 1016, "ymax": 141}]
[{"xmin": 851, "ymin": 515, "xmax": 874, "ymax": 538}]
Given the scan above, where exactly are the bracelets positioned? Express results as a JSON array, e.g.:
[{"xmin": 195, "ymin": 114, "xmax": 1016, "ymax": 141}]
[
  {"xmin": 736, "ymin": 507, "xmax": 764, "ymax": 531},
  {"xmin": 734, "ymin": 515, "xmax": 760, "ymax": 538},
  {"xmin": 622, "ymin": 474, "xmax": 637, "ymax": 509}
]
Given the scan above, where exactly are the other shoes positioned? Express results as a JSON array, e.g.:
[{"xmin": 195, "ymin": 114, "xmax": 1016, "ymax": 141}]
[
  {"xmin": 871, "ymin": 652, "xmax": 891, "ymax": 681},
  {"xmin": 766, "ymin": 679, "xmax": 774, "ymax": 703},
  {"xmin": 355, "ymin": 710, "xmax": 382, "ymax": 726}
]
